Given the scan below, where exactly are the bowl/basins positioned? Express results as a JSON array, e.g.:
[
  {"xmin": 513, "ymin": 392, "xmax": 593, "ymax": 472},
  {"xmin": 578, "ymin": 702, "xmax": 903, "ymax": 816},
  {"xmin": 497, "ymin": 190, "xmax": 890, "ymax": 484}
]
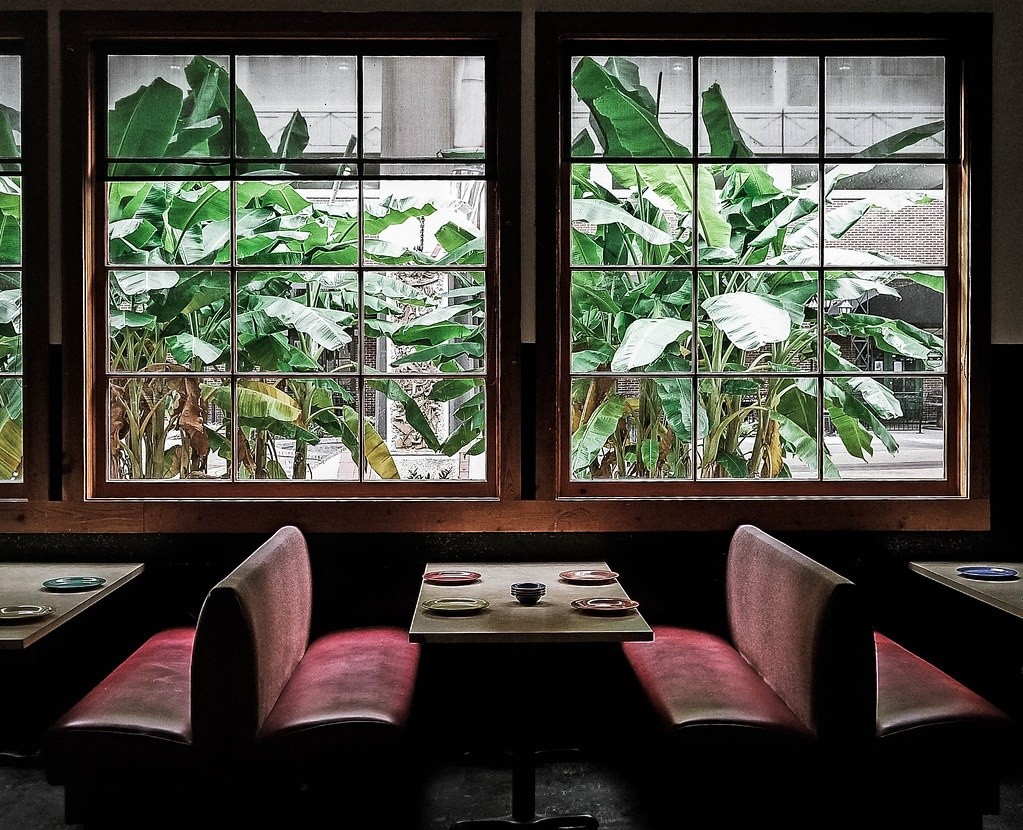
[{"xmin": 509, "ymin": 582, "xmax": 547, "ymax": 606}]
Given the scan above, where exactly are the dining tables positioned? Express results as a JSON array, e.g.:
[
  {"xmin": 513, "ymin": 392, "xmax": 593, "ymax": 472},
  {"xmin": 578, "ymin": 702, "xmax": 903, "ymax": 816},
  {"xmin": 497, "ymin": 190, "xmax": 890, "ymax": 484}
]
[
  {"xmin": 909, "ymin": 559, "xmax": 1022, "ymax": 812},
  {"xmin": 1, "ymin": 560, "xmax": 150, "ymax": 767},
  {"xmin": 413, "ymin": 560, "xmax": 655, "ymax": 830}
]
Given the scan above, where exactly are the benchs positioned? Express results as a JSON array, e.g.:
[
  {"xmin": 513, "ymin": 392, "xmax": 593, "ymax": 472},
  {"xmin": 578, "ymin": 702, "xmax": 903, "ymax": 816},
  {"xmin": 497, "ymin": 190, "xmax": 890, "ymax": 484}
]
[
  {"xmin": 43, "ymin": 519, "xmax": 425, "ymax": 830},
  {"xmin": 618, "ymin": 523, "xmax": 1011, "ymax": 829}
]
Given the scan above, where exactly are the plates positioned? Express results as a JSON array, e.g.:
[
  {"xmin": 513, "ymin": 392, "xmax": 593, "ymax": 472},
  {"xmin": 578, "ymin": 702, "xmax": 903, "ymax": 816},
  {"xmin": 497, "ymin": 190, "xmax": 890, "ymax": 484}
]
[
  {"xmin": 0, "ymin": 604, "xmax": 56, "ymax": 621},
  {"xmin": 421, "ymin": 568, "xmax": 482, "ymax": 586},
  {"xmin": 43, "ymin": 576, "xmax": 105, "ymax": 590},
  {"xmin": 559, "ymin": 568, "xmax": 620, "ymax": 583},
  {"xmin": 571, "ymin": 597, "xmax": 638, "ymax": 615},
  {"xmin": 421, "ymin": 597, "xmax": 489, "ymax": 614},
  {"xmin": 955, "ymin": 565, "xmax": 1019, "ymax": 580}
]
